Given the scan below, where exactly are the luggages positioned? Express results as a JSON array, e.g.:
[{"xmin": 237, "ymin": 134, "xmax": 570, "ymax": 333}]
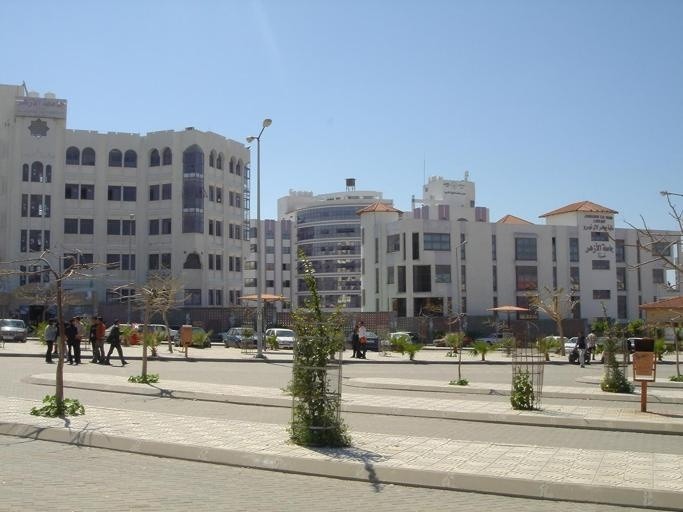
[{"xmin": 569, "ymin": 346, "xmax": 592, "ymax": 363}]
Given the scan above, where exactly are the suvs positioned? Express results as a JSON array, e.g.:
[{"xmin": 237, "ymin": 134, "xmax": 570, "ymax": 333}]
[{"xmin": 475, "ymin": 332, "xmax": 514, "ymax": 346}]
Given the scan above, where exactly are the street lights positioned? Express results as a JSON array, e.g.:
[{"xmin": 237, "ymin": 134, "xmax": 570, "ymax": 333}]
[
  {"xmin": 127, "ymin": 214, "xmax": 135, "ymax": 324},
  {"xmin": 455, "ymin": 241, "xmax": 468, "ymax": 314},
  {"xmin": 246, "ymin": 118, "xmax": 272, "ymax": 358}
]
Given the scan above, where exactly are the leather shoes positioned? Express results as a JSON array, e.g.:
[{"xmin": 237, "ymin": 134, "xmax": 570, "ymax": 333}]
[{"xmin": 46, "ymin": 358, "xmax": 130, "ymax": 366}]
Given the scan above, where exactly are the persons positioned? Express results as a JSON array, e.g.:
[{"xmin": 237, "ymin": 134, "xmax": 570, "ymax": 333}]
[
  {"xmin": 350, "ymin": 325, "xmax": 360, "ymax": 358},
  {"xmin": 354, "ymin": 321, "xmax": 368, "ymax": 360},
  {"xmin": 130, "ymin": 321, "xmax": 140, "ymax": 345},
  {"xmin": 44, "ymin": 315, "xmax": 106, "ymax": 364},
  {"xmin": 575, "ymin": 330, "xmax": 597, "ymax": 369},
  {"xmin": 102, "ymin": 319, "xmax": 130, "ymax": 365}
]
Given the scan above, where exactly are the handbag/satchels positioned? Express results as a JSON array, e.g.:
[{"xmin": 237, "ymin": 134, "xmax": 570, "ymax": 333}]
[{"xmin": 106, "ymin": 334, "xmax": 120, "ymax": 345}]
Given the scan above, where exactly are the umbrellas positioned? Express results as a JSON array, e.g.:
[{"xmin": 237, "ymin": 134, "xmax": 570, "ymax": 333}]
[
  {"xmin": 486, "ymin": 305, "xmax": 529, "ymax": 327},
  {"xmin": 237, "ymin": 294, "xmax": 286, "ymax": 306}
]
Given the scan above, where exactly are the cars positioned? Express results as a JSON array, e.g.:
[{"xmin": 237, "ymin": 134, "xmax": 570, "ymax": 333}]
[
  {"xmin": 432, "ymin": 333, "xmax": 474, "ymax": 347},
  {"xmin": 223, "ymin": 327, "xmax": 257, "ymax": 348},
  {"xmin": 0, "ymin": 319, "xmax": 28, "ymax": 342},
  {"xmin": 345, "ymin": 331, "xmax": 427, "ymax": 351},
  {"xmin": 536, "ymin": 335, "xmax": 643, "ymax": 353},
  {"xmin": 265, "ymin": 328, "xmax": 297, "ymax": 350},
  {"xmin": 105, "ymin": 324, "xmax": 227, "ymax": 348},
  {"xmin": 662, "ymin": 335, "xmax": 676, "ymax": 351}
]
